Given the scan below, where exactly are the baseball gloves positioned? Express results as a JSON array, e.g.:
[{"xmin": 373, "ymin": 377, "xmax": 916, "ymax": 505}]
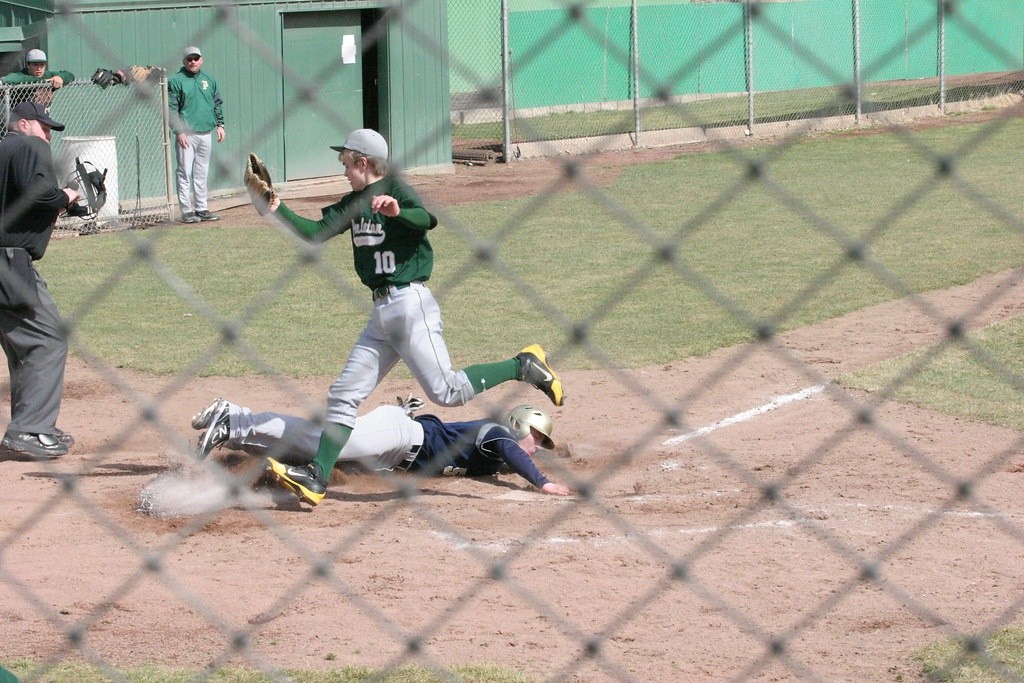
[
  {"xmin": 147, "ymin": 65, "xmax": 163, "ymax": 86},
  {"xmin": 129, "ymin": 65, "xmax": 151, "ymax": 84},
  {"xmin": 92, "ymin": 68, "xmax": 113, "ymax": 89},
  {"xmin": 243, "ymin": 150, "xmax": 274, "ymax": 216}
]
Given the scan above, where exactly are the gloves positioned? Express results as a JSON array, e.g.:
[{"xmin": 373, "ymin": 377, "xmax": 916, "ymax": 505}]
[{"xmin": 92, "ymin": 70, "xmax": 113, "ymax": 87}]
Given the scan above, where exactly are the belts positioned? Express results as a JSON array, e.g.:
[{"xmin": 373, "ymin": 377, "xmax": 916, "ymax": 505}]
[
  {"xmin": 373, "ymin": 283, "xmax": 410, "ymax": 303},
  {"xmin": 398, "ymin": 445, "xmax": 420, "ymax": 469}
]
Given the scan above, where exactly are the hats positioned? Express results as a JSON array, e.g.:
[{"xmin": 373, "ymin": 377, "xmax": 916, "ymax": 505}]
[
  {"xmin": 330, "ymin": 129, "xmax": 388, "ymax": 160},
  {"xmin": 183, "ymin": 47, "xmax": 201, "ymax": 58},
  {"xmin": 25, "ymin": 49, "xmax": 48, "ymax": 63},
  {"xmin": 9, "ymin": 102, "xmax": 65, "ymax": 131},
  {"xmin": 503, "ymin": 405, "xmax": 555, "ymax": 449}
]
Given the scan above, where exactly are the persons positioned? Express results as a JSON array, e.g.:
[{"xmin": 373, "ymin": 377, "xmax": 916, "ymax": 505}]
[
  {"xmin": 0, "ymin": 102, "xmax": 80, "ymax": 460},
  {"xmin": 190, "ymin": 397, "xmax": 570, "ymax": 497},
  {"xmin": 244, "ymin": 128, "xmax": 565, "ymax": 507},
  {"xmin": 167, "ymin": 47, "xmax": 226, "ymax": 223},
  {"xmin": 0, "ymin": 49, "xmax": 75, "ymax": 140}
]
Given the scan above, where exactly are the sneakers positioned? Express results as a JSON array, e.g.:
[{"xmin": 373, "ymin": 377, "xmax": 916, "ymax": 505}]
[
  {"xmin": 1, "ymin": 427, "xmax": 74, "ymax": 457},
  {"xmin": 181, "ymin": 213, "xmax": 201, "ymax": 223},
  {"xmin": 195, "ymin": 210, "xmax": 217, "ymax": 220},
  {"xmin": 195, "ymin": 401, "xmax": 230, "ymax": 461},
  {"xmin": 191, "ymin": 396, "xmax": 225, "ymax": 430},
  {"xmin": 266, "ymin": 457, "xmax": 327, "ymax": 506},
  {"xmin": 515, "ymin": 344, "xmax": 563, "ymax": 407}
]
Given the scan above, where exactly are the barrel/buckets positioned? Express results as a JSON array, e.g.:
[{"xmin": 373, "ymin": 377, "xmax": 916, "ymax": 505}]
[{"xmin": 59, "ymin": 135, "xmax": 118, "ymax": 220}]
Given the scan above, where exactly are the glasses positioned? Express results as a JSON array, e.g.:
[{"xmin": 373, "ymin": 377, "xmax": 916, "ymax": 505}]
[{"xmin": 187, "ymin": 57, "xmax": 199, "ymax": 62}]
